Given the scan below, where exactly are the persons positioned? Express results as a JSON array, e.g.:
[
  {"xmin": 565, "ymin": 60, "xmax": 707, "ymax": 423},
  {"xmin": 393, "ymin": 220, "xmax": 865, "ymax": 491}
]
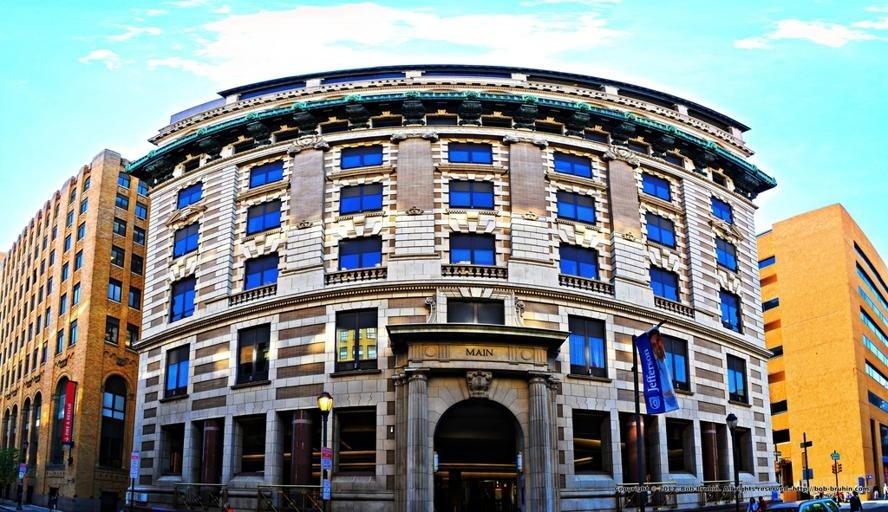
[
  {"xmin": 745, "ymin": 496, "xmax": 759, "ymax": 511},
  {"xmin": 882, "ymin": 482, "xmax": 888, "ymax": 500},
  {"xmin": 758, "ymin": 495, "xmax": 769, "ymax": 511},
  {"xmin": 850, "ymin": 490, "xmax": 864, "ymax": 512},
  {"xmin": 754, "ymin": 506, "xmax": 763, "ymax": 511},
  {"xmin": 818, "ymin": 490, "xmax": 853, "ymax": 503},
  {"xmin": 872, "ymin": 482, "xmax": 879, "ymax": 499}
]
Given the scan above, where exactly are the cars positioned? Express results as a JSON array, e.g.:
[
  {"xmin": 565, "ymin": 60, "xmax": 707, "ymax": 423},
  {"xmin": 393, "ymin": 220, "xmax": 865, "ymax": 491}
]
[{"xmin": 768, "ymin": 498, "xmax": 842, "ymax": 512}]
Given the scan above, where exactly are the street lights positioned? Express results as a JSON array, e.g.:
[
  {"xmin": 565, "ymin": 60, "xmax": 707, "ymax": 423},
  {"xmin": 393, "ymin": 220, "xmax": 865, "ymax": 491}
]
[
  {"xmin": 725, "ymin": 413, "xmax": 740, "ymax": 512},
  {"xmin": 16, "ymin": 440, "xmax": 29, "ymax": 511},
  {"xmin": 317, "ymin": 391, "xmax": 333, "ymax": 511}
]
